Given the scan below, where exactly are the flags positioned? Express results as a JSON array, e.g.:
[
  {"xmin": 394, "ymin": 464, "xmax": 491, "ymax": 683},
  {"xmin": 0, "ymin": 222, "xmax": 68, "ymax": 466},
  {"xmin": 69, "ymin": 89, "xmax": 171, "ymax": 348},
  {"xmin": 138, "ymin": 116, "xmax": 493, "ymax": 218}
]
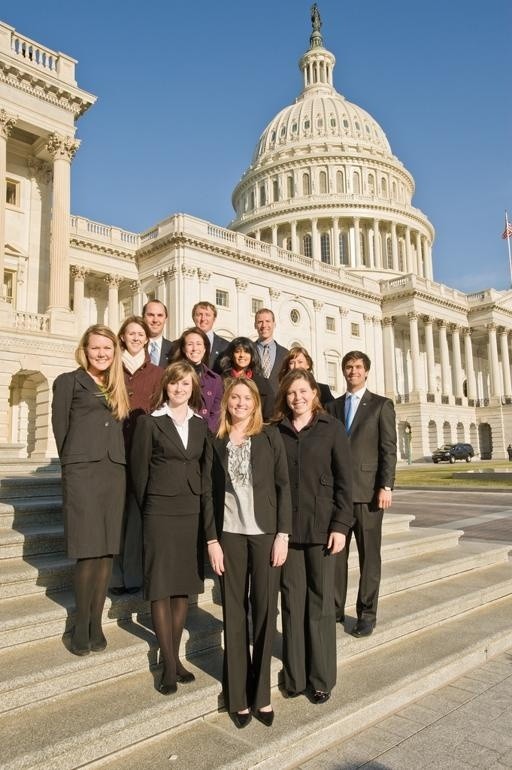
[{"xmin": 500, "ymin": 216, "xmax": 512, "ymax": 241}]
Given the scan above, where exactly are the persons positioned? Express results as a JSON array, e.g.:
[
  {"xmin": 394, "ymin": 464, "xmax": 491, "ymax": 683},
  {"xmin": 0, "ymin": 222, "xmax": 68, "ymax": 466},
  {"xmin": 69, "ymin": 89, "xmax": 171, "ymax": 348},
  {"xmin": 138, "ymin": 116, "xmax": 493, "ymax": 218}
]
[
  {"xmin": 174, "ymin": 326, "xmax": 228, "ymax": 440},
  {"xmin": 171, "ymin": 300, "xmax": 234, "ymax": 371},
  {"xmin": 106, "ymin": 316, "xmax": 166, "ymax": 599},
  {"xmin": 201, "ymin": 371, "xmax": 293, "ymax": 731},
  {"xmin": 140, "ymin": 298, "xmax": 174, "ymax": 369},
  {"xmin": 49, "ymin": 322, "xmax": 128, "ymax": 658},
  {"xmin": 267, "ymin": 370, "xmax": 358, "ymax": 703},
  {"xmin": 278, "ymin": 347, "xmax": 336, "ymax": 413},
  {"xmin": 249, "ymin": 307, "xmax": 295, "ymax": 401},
  {"xmin": 216, "ymin": 334, "xmax": 277, "ymax": 421},
  {"xmin": 327, "ymin": 348, "xmax": 396, "ymax": 638},
  {"xmin": 128, "ymin": 360, "xmax": 209, "ymax": 695}
]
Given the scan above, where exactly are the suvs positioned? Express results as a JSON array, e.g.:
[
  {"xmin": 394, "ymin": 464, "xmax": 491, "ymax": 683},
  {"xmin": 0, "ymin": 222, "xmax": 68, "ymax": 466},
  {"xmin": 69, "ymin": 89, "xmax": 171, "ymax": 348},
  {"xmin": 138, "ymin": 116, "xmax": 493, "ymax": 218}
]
[{"xmin": 432, "ymin": 442, "xmax": 475, "ymax": 465}]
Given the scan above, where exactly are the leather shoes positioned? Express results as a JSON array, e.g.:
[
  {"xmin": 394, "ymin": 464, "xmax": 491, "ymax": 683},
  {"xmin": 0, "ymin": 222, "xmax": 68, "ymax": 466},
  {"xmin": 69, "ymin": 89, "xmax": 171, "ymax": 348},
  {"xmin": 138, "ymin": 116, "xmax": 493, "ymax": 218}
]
[
  {"xmin": 90, "ymin": 624, "xmax": 107, "ymax": 654},
  {"xmin": 176, "ymin": 662, "xmax": 196, "ymax": 686},
  {"xmin": 285, "ymin": 684, "xmax": 333, "ymax": 705},
  {"xmin": 157, "ymin": 663, "xmax": 177, "ymax": 696},
  {"xmin": 231, "ymin": 700, "xmax": 252, "ymax": 730},
  {"xmin": 254, "ymin": 705, "xmax": 274, "ymax": 729},
  {"xmin": 351, "ymin": 610, "xmax": 378, "ymax": 638},
  {"xmin": 69, "ymin": 622, "xmax": 90, "ymax": 656}
]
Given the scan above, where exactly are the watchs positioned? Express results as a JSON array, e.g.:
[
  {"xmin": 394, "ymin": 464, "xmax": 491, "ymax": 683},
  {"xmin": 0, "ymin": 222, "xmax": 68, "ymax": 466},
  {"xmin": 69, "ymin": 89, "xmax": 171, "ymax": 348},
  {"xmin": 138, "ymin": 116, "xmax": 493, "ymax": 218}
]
[{"xmin": 380, "ymin": 486, "xmax": 394, "ymax": 492}]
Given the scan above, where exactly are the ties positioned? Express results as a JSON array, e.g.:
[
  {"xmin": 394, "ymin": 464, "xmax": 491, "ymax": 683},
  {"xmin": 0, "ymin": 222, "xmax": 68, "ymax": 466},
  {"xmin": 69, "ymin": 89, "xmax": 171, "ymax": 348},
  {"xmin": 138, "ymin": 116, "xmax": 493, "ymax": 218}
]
[
  {"xmin": 148, "ymin": 342, "xmax": 160, "ymax": 366},
  {"xmin": 260, "ymin": 344, "xmax": 272, "ymax": 378},
  {"xmin": 345, "ymin": 395, "xmax": 357, "ymax": 434}
]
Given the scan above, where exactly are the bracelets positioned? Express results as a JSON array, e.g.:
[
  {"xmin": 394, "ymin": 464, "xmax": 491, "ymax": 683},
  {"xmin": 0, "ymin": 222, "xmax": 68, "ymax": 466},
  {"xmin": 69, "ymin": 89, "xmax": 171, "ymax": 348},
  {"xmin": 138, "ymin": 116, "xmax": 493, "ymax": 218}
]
[
  {"xmin": 206, "ymin": 539, "xmax": 220, "ymax": 546},
  {"xmin": 278, "ymin": 533, "xmax": 291, "ymax": 543}
]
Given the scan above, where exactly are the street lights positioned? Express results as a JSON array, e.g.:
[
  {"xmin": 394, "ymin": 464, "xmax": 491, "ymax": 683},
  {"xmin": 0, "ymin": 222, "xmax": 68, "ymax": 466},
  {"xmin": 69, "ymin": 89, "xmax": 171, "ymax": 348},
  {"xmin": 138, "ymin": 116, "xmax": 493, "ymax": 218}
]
[{"xmin": 404, "ymin": 427, "xmax": 413, "ymax": 466}]
